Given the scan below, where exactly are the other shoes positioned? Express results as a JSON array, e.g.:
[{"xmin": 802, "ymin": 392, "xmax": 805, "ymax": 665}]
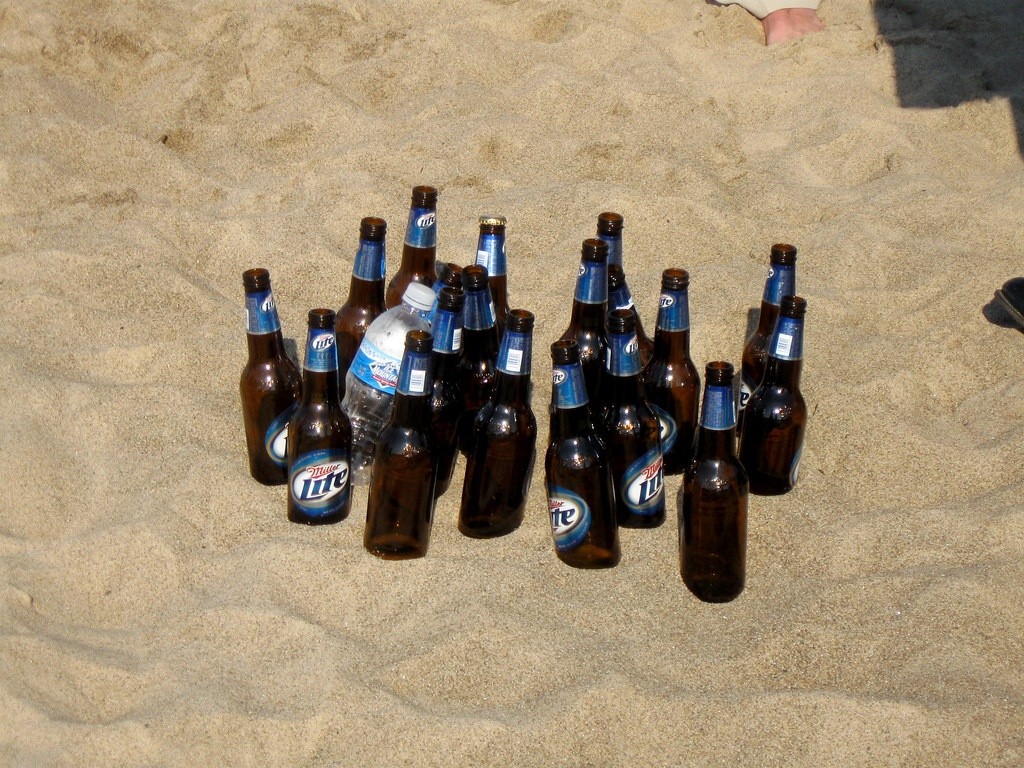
[{"xmin": 993, "ymin": 276, "xmax": 1024, "ymax": 327}]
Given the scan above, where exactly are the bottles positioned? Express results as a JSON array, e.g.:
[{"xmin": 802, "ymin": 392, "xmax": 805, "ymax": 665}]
[
  {"xmin": 239, "ymin": 266, "xmax": 301, "ymax": 487},
  {"xmin": 542, "ymin": 210, "xmax": 700, "ymax": 572},
  {"xmin": 736, "ymin": 241, "xmax": 809, "ymax": 499},
  {"xmin": 289, "ymin": 181, "xmax": 538, "ymax": 563},
  {"xmin": 680, "ymin": 360, "xmax": 747, "ymax": 605}
]
